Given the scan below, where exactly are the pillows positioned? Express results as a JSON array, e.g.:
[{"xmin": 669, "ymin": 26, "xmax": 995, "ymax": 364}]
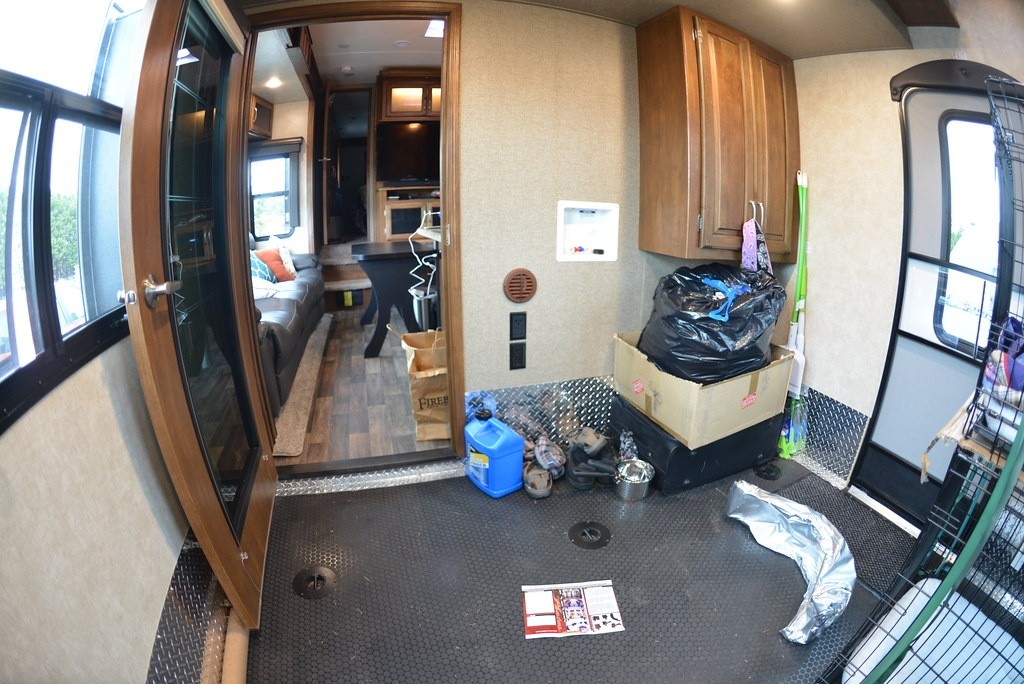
[{"xmin": 245, "ymin": 235, "xmax": 298, "ymax": 301}]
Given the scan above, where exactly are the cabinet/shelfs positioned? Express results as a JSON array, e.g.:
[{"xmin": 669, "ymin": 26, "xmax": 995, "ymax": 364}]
[
  {"xmin": 247, "ymin": 92, "xmax": 273, "ymax": 141},
  {"xmin": 635, "ymin": 6, "xmax": 801, "ymax": 264},
  {"xmin": 365, "ymin": 69, "xmax": 441, "ymax": 243}
]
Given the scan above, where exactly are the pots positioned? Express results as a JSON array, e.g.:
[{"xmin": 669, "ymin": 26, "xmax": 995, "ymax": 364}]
[{"xmin": 571, "ymin": 458, "xmax": 655, "ymax": 501}]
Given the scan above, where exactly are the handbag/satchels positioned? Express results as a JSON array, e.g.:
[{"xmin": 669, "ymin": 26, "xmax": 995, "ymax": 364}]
[{"xmin": 386, "ymin": 321, "xmax": 452, "ymax": 441}]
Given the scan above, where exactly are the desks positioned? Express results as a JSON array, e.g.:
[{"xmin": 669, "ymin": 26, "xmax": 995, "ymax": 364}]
[{"xmin": 351, "ymin": 240, "xmax": 438, "ymax": 359}]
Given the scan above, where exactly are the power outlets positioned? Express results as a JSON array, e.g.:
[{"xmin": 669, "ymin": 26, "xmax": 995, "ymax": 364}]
[
  {"xmin": 509, "ymin": 342, "xmax": 526, "ymax": 370},
  {"xmin": 509, "ymin": 312, "xmax": 526, "ymax": 341}
]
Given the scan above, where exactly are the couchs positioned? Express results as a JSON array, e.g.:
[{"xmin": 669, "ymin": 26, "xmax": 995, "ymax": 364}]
[{"xmin": 248, "ymin": 232, "xmax": 326, "ymax": 419}]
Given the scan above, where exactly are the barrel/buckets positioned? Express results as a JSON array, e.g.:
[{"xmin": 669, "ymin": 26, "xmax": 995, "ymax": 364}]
[{"xmin": 463, "ymin": 409, "xmax": 524, "ymax": 499}]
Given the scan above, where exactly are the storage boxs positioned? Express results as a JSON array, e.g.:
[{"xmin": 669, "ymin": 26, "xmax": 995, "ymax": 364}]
[{"xmin": 606, "ymin": 331, "xmax": 795, "ymax": 451}]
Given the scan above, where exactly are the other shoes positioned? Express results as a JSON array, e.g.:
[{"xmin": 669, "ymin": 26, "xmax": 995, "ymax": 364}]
[{"xmin": 523, "ymin": 427, "xmax": 620, "ymax": 499}]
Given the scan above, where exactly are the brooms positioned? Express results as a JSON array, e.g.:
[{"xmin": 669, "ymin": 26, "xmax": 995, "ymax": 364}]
[{"xmin": 776, "ymin": 170, "xmax": 809, "ymax": 460}]
